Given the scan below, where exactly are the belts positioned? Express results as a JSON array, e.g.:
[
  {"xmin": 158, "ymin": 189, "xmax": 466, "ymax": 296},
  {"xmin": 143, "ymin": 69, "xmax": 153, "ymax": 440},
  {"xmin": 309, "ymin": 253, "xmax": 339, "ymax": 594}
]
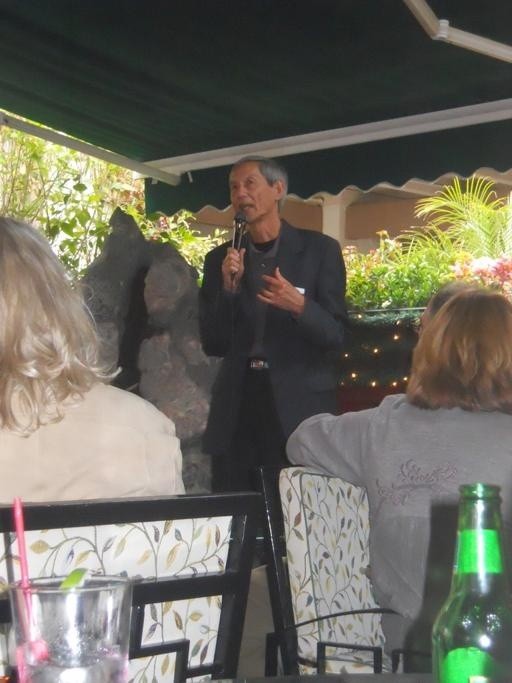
[{"xmin": 246, "ymin": 359, "xmax": 268, "ymax": 371}]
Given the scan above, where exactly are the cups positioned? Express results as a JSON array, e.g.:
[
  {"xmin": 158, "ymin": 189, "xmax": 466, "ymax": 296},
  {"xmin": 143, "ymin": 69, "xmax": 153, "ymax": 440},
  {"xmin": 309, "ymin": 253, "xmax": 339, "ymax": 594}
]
[{"xmin": 6, "ymin": 574, "xmax": 134, "ymax": 683}]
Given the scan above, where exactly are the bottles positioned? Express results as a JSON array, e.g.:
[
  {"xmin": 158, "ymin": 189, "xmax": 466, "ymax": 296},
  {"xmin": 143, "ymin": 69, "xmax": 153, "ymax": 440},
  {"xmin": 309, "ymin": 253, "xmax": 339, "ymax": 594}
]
[{"xmin": 429, "ymin": 480, "xmax": 512, "ymax": 683}]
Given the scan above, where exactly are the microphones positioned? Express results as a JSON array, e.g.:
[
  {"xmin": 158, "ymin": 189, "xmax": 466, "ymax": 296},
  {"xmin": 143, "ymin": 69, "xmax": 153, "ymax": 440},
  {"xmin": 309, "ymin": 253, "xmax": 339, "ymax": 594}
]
[{"xmin": 229, "ymin": 210, "xmax": 248, "ymax": 281}]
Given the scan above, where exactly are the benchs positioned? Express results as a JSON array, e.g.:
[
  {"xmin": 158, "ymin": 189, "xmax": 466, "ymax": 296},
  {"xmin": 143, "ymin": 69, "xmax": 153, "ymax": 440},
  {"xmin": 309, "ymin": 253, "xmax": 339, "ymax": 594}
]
[
  {"xmin": 0, "ymin": 491, "xmax": 259, "ymax": 682},
  {"xmin": 255, "ymin": 461, "xmax": 435, "ymax": 675}
]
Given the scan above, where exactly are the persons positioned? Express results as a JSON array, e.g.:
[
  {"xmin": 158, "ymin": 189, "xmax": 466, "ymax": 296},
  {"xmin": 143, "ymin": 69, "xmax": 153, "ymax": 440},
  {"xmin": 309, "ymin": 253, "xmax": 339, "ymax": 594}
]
[
  {"xmin": 200, "ymin": 154, "xmax": 346, "ymax": 494},
  {"xmin": 286, "ymin": 281, "xmax": 512, "ymax": 672},
  {"xmin": 0, "ymin": 214, "xmax": 188, "ymax": 504}
]
